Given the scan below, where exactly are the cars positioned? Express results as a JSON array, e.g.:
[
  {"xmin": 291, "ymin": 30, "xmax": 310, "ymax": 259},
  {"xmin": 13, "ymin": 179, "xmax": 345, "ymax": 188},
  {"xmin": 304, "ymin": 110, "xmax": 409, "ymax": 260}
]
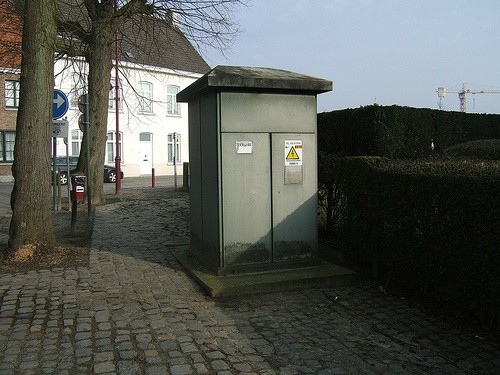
[{"xmin": 50, "ymin": 156, "xmax": 123, "ymax": 185}]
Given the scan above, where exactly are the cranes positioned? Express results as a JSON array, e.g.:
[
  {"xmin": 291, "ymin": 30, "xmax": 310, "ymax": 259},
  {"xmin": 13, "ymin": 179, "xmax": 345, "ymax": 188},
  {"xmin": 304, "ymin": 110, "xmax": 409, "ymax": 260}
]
[{"xmin": 437, "ymin": 85, "xmax": 499, "ymax": 113}]
